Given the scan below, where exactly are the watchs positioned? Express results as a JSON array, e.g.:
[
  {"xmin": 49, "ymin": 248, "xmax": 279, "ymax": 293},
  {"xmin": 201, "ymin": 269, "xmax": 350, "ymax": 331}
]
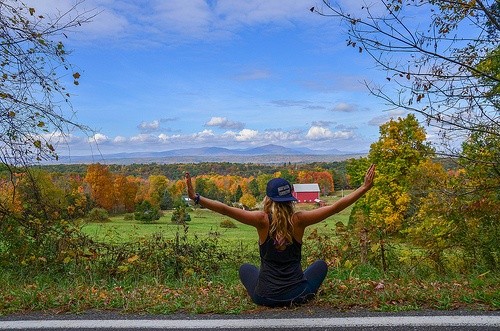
[{"xmin": 193, "ymin": 193, "xmax": 201, "ymax": 206}]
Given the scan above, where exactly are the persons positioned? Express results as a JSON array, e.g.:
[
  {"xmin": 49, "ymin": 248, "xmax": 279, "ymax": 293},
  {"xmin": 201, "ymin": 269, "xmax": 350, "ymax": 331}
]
[{"xmin": 184, "ymin": 164, "xmax": 376, "ymax": 311}]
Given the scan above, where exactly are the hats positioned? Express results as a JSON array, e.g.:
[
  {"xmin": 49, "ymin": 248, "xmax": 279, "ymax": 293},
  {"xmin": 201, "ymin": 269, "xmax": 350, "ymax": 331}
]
[{"xmin": 266, "ymin": 177, "xmax": 297, "ymax": 202}]
